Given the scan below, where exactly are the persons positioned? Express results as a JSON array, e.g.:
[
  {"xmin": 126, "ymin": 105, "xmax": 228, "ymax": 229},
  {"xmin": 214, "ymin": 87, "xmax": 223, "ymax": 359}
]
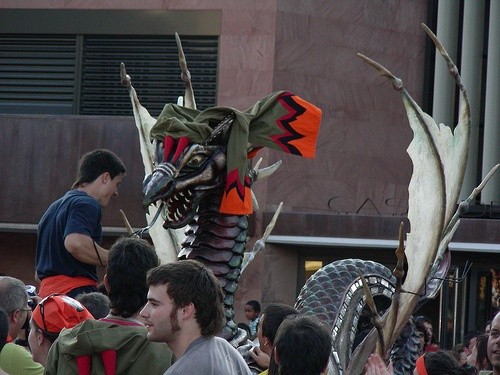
[
  {"xmin": 34, "ymin": 150, "xmax": 126, "ymax": 298},
  {"xmin": 0, "ymin": 237, "xmax": 500, "ymax": 375}
]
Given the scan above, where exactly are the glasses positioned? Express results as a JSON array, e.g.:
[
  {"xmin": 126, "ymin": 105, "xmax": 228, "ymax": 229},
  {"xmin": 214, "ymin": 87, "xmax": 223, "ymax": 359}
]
[
  {"xmin": 8, "ymin": 306, "xmax": 32, "ymax": 319},
  {"xmin": 38, "ymin": 293, "xmax": 66, "ymax": 332}
]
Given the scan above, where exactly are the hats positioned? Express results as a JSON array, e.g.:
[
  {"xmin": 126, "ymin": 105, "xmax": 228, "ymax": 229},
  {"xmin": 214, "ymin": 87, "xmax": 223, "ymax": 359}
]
[{"xmin": 32, "ymin": 293, "xmax": 96, "ymax": 334}]
